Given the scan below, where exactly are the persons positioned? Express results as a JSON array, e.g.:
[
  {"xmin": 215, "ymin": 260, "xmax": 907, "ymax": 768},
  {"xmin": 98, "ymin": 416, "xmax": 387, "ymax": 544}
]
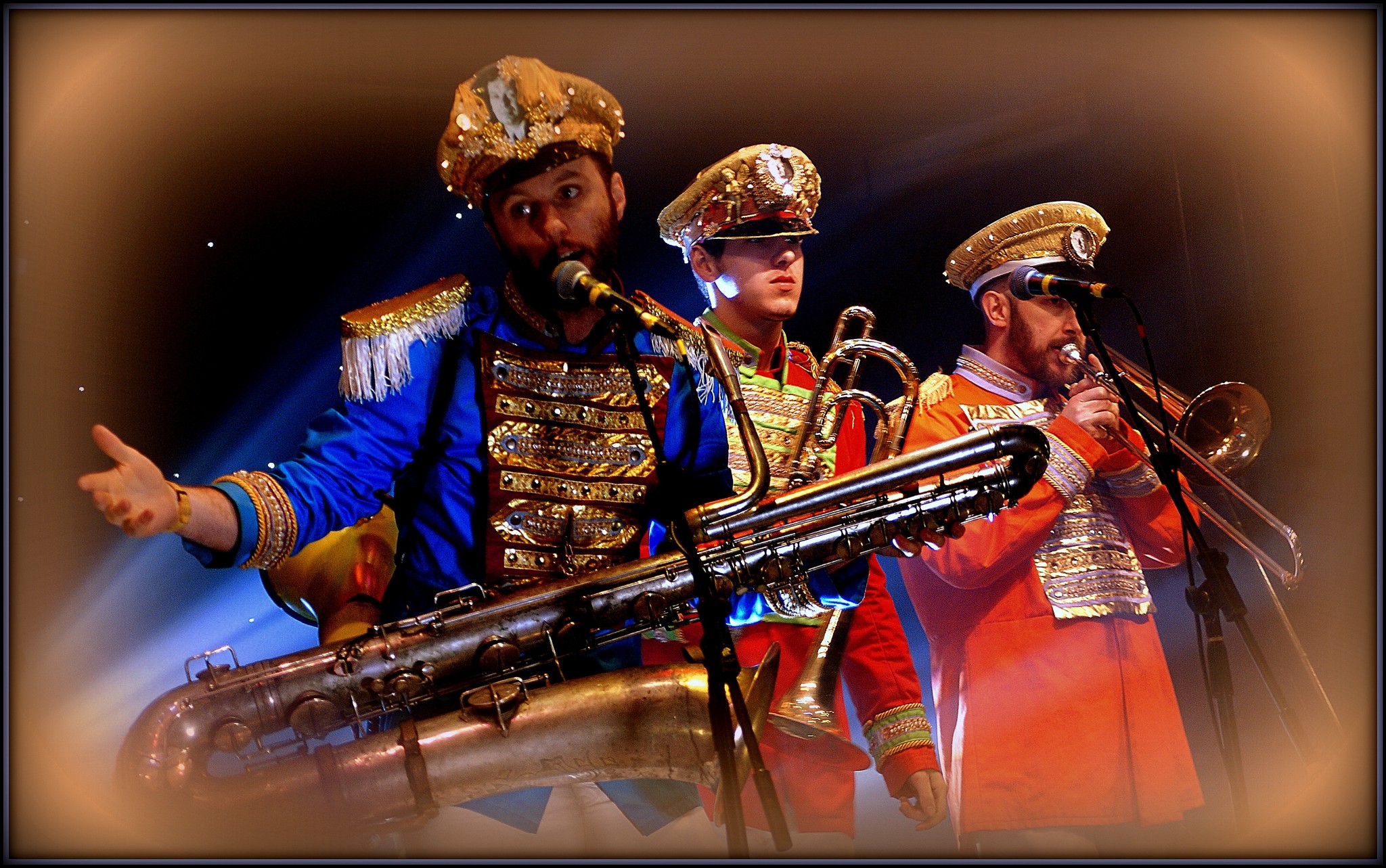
[
  {"xmin": 656, "ymin": 139, "xmax": 953, "ymax": 858},
  {"xmin": 863, "ymin": 195, "xmax": 1206, "ymax": 858},
  {"xmin": 70, "ymin": 53, "xmax": 970, "ymax": 858}
]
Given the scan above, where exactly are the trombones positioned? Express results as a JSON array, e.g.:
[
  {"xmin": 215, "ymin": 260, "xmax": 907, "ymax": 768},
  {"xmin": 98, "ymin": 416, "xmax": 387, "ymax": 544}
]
[
  {"xmin": 760, "ymin": 302, "xmax": 917, "ymax": 775},
  {"xmin": 1045, "ymin": 333, "xmax": 1304, "ymax": 587}
]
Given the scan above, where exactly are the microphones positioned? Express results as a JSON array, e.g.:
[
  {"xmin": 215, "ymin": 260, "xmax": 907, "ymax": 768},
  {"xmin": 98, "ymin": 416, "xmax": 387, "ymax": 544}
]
[
  {"xmin": 1008, "ymin": 265, "xmax": 1117, "ymax": 302},
  {"xmin": 552, "ymin": 260, "xmax": 682, "ymax": 343}
]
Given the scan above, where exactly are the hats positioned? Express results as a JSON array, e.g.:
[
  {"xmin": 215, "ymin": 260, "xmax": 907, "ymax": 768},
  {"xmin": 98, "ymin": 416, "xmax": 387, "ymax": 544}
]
[
  {"xmin": 657, "ymin": 144, "xmax": 821, "ymax": 263},
  {"xmin": 942, "ymin": 200, "xmax": 1111, "ymax": 310},
  {"xmin": 436, "ymin": 55, "xmax": 624, "ymax": 209}
]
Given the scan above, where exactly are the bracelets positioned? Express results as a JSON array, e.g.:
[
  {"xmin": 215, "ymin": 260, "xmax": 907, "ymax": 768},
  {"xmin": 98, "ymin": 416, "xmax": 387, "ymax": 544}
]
[{"xmin": 162, "ymin": 478, "xmax": 193, "ymax": 534}]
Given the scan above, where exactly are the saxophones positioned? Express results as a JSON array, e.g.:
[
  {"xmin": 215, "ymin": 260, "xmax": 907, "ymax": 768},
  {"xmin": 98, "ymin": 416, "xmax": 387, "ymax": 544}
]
[{"xmin": 110, "ymin": 324, "xmax": 1051, "ymax": 849}]
